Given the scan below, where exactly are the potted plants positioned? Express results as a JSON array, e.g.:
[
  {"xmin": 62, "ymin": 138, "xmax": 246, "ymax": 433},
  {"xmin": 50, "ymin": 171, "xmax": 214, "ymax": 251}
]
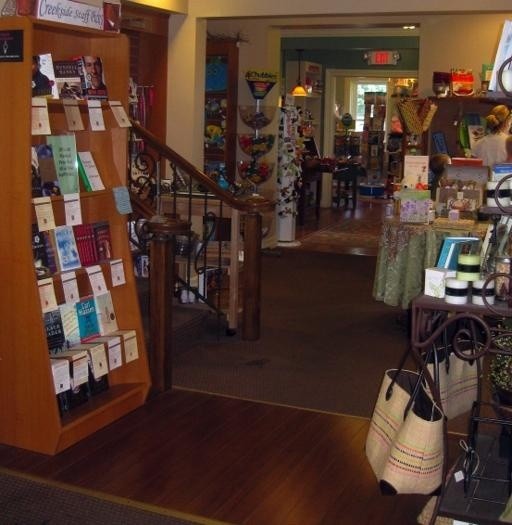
[{"xmin": 481, "ymin": 317, "xmax": 511, "ymax": 458}]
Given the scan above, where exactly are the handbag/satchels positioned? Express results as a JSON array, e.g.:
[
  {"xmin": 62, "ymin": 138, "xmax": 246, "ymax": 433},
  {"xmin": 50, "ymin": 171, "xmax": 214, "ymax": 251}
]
[{"xmin": 365, "ymin": 368, "xmax": 445, "ymax": 495}]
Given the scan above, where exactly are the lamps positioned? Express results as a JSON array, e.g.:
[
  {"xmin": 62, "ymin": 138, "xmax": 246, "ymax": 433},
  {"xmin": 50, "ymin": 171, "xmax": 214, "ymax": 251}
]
[
  {"xmin": 291, "ymin": 48, "xmax": 307, "ymax": 96},
  {"xmin": 362, "ymin": 50, "xmax": 400, "ymax": 66}
]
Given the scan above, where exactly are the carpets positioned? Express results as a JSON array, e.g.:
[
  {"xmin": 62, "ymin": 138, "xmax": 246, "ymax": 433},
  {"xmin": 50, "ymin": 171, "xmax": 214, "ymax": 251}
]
[
  {"xmin": 1, "ymin": 470, "xmax": 230, "ymax": 524},
  {"xmin": 170, "ymin": 248, "xmax": 471, "ymax": 438}
]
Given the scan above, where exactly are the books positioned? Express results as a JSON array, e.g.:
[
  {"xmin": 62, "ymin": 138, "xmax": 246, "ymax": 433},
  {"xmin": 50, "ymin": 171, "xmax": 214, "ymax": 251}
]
[
  {"xmin": 113, "ymin": 131, "xmax": 158, "ymax": 281},
  {"xmin": 198, "ymin": 267, "xmax": 229, "ymax": 309},
  {"xmin": 431, "ymin": 213, "xmax": 511, "ymax": 271},
  {"xmin": 403, "ymin": 154, "xmax": 429, "ymax": 188},
  {"xmin": 398, "ymin": 188, "xmax": 431, "ymax": 226},
  {"xmin": 30, "ymin": 52, "xmax": 120, "ymax": 417}
]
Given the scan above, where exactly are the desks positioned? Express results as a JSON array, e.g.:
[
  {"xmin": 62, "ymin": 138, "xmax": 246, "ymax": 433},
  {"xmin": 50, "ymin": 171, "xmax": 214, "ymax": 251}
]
[
  {"xmin": 410, "ymin": 283, "xmax": 512, "ymax": 524},
  {"xmin": 375, "ymin": 210, "xmax": 492, "ymax": 311}
]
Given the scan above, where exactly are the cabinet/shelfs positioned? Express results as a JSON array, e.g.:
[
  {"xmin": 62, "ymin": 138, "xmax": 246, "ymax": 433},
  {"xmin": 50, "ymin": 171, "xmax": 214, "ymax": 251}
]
[
  {"xmin": 159, "ymin": 188, "xmax": 246, "ymax": 336},
  {"xmin": 1, "ymin": 13, "xmax": 153, "ymax": 457},
  {"xmin": 205, "ymin": 37, "xmax": 238, "ymax": 188},
  {"xmin": 285, "ymin": 61, "xmax": 324, "ymax": 156}
]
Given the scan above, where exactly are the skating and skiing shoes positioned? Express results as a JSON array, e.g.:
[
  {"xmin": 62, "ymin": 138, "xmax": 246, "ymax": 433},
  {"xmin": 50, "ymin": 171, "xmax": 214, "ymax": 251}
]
[{"xmin": 422, "ymin": 341, "xmax": 483, "ymax": 421}]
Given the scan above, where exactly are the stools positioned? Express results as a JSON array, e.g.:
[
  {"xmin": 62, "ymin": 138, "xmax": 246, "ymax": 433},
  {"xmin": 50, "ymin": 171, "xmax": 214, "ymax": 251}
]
[
  {"xmin": 336, "ymin": 162, "xmax": 360, "ymax": 210},
  {"xmin": 296, "ymin": 173, "xmax": 320, "ymax": 226}
]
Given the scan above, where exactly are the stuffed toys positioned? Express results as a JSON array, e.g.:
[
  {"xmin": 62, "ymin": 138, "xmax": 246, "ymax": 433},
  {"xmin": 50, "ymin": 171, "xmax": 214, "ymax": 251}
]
[{"xmin": 204, "ymin": 123, "xmax": 226, "ymax": 151}]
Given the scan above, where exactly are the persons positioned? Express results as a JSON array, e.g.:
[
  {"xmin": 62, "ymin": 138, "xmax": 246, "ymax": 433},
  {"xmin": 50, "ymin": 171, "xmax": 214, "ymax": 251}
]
[
  {"xmin": 428, "ymin": 154, "xmax": 455, "ymax": 203},
  {"xmin": 470, "ymin": 103, "xmax": 512, "ymax": 167}
]
